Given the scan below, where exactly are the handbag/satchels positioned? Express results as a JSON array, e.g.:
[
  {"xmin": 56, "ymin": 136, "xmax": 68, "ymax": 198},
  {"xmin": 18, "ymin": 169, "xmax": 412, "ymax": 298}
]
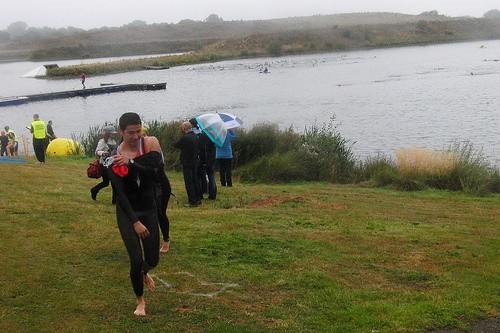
[{"xmin": 87, "ymin": 151, "xmax": 101, "ymax": 179}]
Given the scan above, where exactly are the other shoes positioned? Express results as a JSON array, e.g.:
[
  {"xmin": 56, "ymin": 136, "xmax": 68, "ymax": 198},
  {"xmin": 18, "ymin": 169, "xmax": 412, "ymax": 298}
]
[
  {"xmin": 184, "ymin": 202, "xmax": 197, "ymax": 207},
  {"xmin": 91, "ymin": 190, "xmax": 96, "ymax": 200}
]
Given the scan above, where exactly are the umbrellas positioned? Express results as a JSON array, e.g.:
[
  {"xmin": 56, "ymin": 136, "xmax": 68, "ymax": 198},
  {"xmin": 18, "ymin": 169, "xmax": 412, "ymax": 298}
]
[
  {"xmin": 196, "ymin": 113, "xmax": 227, "ymax": 147},
  {"xmin": 215, "ymin": 111, "xmax": 244, "ymax": 130}
]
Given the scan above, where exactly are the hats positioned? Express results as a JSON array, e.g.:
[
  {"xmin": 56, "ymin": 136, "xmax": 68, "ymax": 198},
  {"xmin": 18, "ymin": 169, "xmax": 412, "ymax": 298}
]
[{"xmin": 104, "ymin": 125, "xmax": 117, "ymax": 134}]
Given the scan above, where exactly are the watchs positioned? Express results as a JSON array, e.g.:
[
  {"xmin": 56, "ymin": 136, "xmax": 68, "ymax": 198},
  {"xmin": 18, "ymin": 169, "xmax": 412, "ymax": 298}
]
[{"xmin": 128, "ymin": 159, "xmax": 135, "ymax": 167}]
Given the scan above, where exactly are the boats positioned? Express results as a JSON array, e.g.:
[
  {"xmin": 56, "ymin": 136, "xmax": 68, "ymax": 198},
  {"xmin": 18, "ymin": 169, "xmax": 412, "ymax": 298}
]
[{"xmin": 141, "ymin": 66, "xmax": 170, "ymax": 70}]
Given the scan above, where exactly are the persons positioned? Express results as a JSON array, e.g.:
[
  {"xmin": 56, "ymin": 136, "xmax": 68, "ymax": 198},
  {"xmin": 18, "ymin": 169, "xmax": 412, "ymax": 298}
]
[
  {"xmin": 0, "ymin": 126, "xmax": 18, "ymax": 157},
  {"xmin": 91, "ymin": 112, "xmax": 172, "ymax": 316},
  {"xmin": 216, "ymin": 130, "xmax": 236, "ymax": 187},
  {"xmin": 80, "ymin": 72, "xmax": 86, "ymax": 88},
  {"xmin": 172, "ymin": 118, "xmax": 217, "ymax": 207},
  {"xmin": 30, "ymin": 114, "xmax": 57, "ymax": 164}
]
